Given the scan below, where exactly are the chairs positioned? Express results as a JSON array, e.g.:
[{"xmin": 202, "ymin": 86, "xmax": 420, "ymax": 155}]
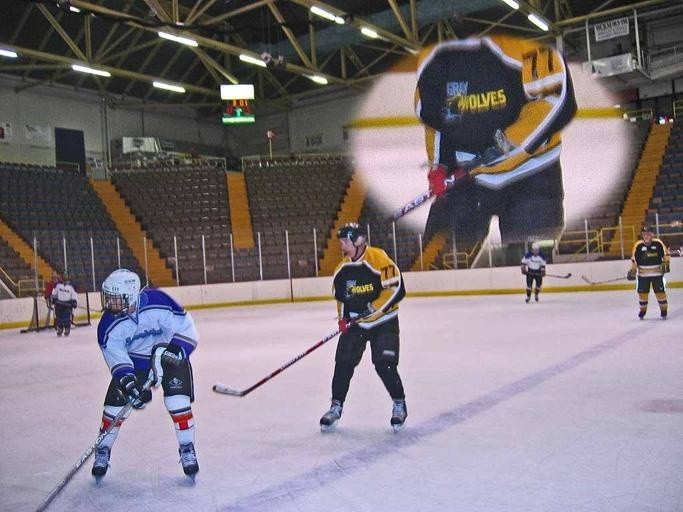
[
  {"xmin": 559, "ymin": 119, "xmax": 681, "ymax": 271},
  {"xmin": 357, "ymin": 194, "xmax": 483, "ymax": 268},
  {"xmin": 0, "ymin": 152, "xmax": 357, "ymax": 298}
]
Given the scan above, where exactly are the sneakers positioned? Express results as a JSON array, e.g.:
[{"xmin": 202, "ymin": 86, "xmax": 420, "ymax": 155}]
[
  {"xmin": 660, "ymin": 309, "xmax": 668, "ymax": 318},
  {"xmin": 319, "ymin": 399, "xmax": 343, "ymax": 426},
  {"xmin": 64, "ymin": 327, "xmax": 70, "ymax": 335},
  {"xmin": 56, "ymin": 328, "xmax": 63, "ymax": 335},
  {"xmin": 638, "ymin": 309, "xmax": 647, "ymax": 318},
  {"xmin": 526, "ymin": 296, "xmax": 531, "ymax": 303},
  {"xmin": 534, "ymin": 295, "xmax": 538, "ymax": 301},
  {"xmin": 389, "ymin": 396, "xmax": 408, "ymax": 426},
  {"xmin": 90, "ymin": 445, "xmax": 112, "ymax": 476},
  {"xmin": 178, "ymin": 442, "xmax": 200, "ymax": 475}
]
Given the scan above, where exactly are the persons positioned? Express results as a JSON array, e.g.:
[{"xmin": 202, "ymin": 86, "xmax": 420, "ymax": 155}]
[
  {"xmin": 628, "ymin": 225, "xmax": 669, "ymax": 318},
  {"xmin": 521, "ymin": 242, "xmax": 545, "ymax": 302},
  {"xmin": 90, "ymin": 268, "xmax": 200, "ymax": 474},
  {"xmin": 51, "ymin": 273, "xmax": 77, "ymax": 336},
  {"xmin": 319, "ymin": 221, "xmax": 408, "ymax": 426},
  {"xmin": 414, "ymin": 38, "xmax": 576, "ymax": 246},
  {"xmin": 44, "ymin": 271, "xmax": 75, "ymax": 329}
]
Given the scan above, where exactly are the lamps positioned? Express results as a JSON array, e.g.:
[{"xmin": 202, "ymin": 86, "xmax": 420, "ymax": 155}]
[
  {"xmin": 152, "ymin": 80, "xmax": 187, "ymax": 94},
  {"xmin": 1, "ymin": 50, "xmax": 20, "ymax": 59},
  {"xmin": 240, "ymin": 53, "xmax": 267, "ymax": 71},
  {"xmin": 71, "ymin": 64, "xmax": 113, "ymax": 78},
  {"xmin": 154, "ymin": 31, "xmax": 199, "ymax": 49},
  {"xmin": 308, "ymin": 6, "xmax": 348, "ymax": 25},
  {"xmin": 360, "ymin": 27, "xmax": 381, "ymax": 41},
  {"xmin": 302, "ymin": 73, "xmax": 330, "ymax": 87},
  {"xmin": 494, "ymin": 0, "xmax": 560, "ymax": 35},
  {"xmin": 55, "ymin": 3, "xmax": 84, "ymax": 15}
]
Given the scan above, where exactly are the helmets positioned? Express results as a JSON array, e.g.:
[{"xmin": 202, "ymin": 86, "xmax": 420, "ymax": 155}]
[
  {"xmin": 100, "ymin": 268, "xmax": 141, "ymax": 306},
  {"xmin": 336, "ymin": 221, "xmax": 366, "ymax": 248},
  {"xmin": 641, "ymin": 225, "xmax": 654, "ymax": 235}
]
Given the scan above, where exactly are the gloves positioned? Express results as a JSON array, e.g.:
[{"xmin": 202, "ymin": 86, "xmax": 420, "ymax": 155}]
[
  {"xmin": 626, "ymin": 270, "xmax": 636, "ymax": 281},
  {"xmin": 660, "ymin": 263, "xmax": 670, "ymax": 273},
  {"xmin": 118, "ymin": 373, "xmax": 152, "ymax": 410},
  {"xmin": 71, "ymin": 299, "xmax": 77, "ymax": 308},
  {"xmin": 160, "ymin": 343, "xmax": 187, "ymax": 370},
  {"xmin": 521, "ymin": 268, "xmax": 527, "ymax": 274},
  {"xmin": 540, "ymin": 270, "xmax": 545, "ymax": 276}
]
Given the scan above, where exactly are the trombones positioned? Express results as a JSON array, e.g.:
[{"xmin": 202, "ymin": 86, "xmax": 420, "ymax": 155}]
[{"xmin": 212, "ymin": 310, "xmax": 356, "ymax": 396}]
